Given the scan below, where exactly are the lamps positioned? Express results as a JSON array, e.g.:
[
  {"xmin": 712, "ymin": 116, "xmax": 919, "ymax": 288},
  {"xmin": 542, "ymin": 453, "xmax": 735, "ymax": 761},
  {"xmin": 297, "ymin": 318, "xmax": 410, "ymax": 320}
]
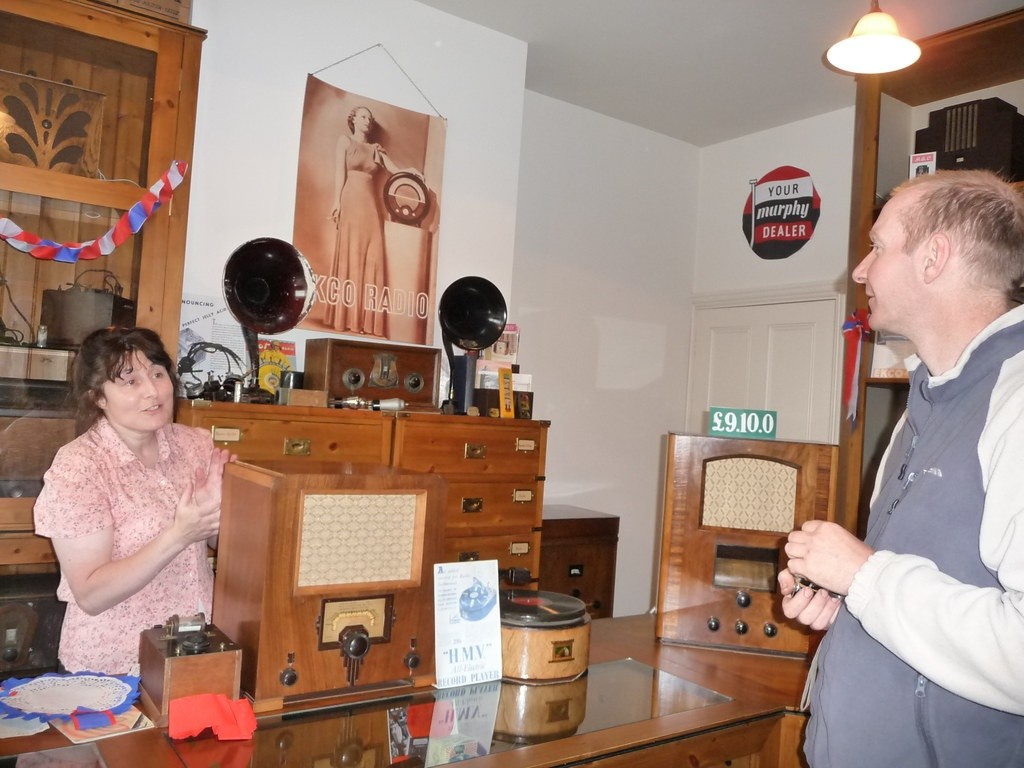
[{"xmin": 826, "ymin": 0, "xmax": 921, "ymax": 75}]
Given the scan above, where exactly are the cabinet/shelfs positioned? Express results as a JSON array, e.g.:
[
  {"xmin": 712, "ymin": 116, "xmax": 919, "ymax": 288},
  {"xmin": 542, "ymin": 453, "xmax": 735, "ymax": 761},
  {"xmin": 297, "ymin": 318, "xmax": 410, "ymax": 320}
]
[
  {"xmin": 0, "ymin": 0, "xmax": 210, "ymax": 680},
  {"xmin": 538, "ymin": 504, "xmax": 620, "ymax": 618}
]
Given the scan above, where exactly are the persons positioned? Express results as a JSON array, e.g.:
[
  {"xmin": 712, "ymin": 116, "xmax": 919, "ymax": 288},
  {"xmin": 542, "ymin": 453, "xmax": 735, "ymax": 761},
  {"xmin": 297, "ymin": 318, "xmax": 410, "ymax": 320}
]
[
  {"xmin": 258, "ymin": 340, "xmax": 292, "ymax": 395},
  {"xmin": 32, "ymin": 327, "xmax": 238, "ymax": 678},
  {"xmin": 779, "ymin": 168, "xmax": 1024, "ymax": 767},
  {"xmin": 324, "ymin": 107, "xmax": 425, "ymax": 340}
]
